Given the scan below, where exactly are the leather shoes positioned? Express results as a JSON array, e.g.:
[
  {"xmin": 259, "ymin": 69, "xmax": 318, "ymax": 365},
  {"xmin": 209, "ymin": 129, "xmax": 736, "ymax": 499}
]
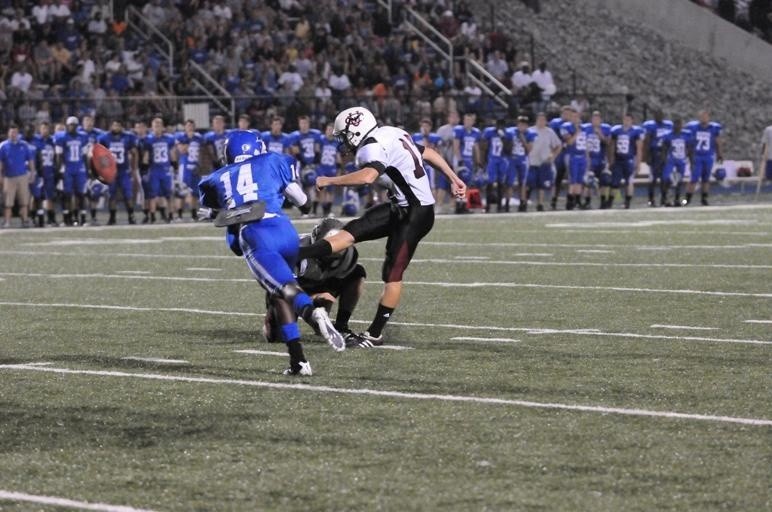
[{"xmin": 498, "ymin": 129, "xmax": 505, "ymax": 138}]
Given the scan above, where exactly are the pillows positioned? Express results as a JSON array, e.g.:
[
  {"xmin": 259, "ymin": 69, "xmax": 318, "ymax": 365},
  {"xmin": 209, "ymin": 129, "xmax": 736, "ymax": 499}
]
[
  {"xmin": 713, "ymin": 167, "xmax": 726, "ymax": 180},
  {"xmin": 300, "ymin": 169, "xmax": 316, "ymax": 186},
  {"xmin": 455, "ymin": 166, "xmax": 472, "ymax": 183},
  {"xmin": 88, "ymin": 178, "xmax": 108, "ymax": 199},
  {"xmin": 310, "ymin": 219, "xmax": 350, "ymax": 271},
  {"xmin": 344, "ymin": 203, "xmax": 357, "ymax": 217},
  {"xmin": 332, "ymin": 107, "xmax": 379, "ymax": 157},
  {"xmin": 66, "ymin": 116, "xmax": 78, "ymax": 128},
  {"xmin": 223, "ymin": 130, "xmax": 267, "ymax": 167},
  {"xmin": 599, "ymin": 170, "xmax": 612, "ymax": 185}
]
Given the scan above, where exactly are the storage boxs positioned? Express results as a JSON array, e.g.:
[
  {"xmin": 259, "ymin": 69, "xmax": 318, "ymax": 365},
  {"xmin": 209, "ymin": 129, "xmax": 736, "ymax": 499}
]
[{"xmin": 493, "ymin": 158, "xmax": 766, "ymax": 202}]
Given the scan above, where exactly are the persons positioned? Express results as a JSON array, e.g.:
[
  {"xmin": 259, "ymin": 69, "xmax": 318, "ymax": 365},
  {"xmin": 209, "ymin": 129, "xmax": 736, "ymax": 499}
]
[
  {"xmin": 300, "ymin": 105, "xmax": 467, "ymax": 349},
  {"xmin": 0, "ymin": 105, "xmax": 723, "ymax": 229},
  {"xmin": 759, "ymin": 126, "xmax": 771, "ymax": 179},
  {"xmin": 263, "ymin": 218, "xmax": 367, "ymax": 343},
  {"xmin": 197, "ymin": 129, "xmax": 347, "ymax": 378},
  {"xmin": 2, "ymin": 3, "xmax": 558, "ymax": 128}
]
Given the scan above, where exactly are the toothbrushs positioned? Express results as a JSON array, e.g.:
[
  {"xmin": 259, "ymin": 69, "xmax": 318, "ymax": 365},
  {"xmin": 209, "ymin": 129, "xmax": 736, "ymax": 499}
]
[{"xmin": 92, "ymin": 143, "xmax": 116, "ymax": 185}]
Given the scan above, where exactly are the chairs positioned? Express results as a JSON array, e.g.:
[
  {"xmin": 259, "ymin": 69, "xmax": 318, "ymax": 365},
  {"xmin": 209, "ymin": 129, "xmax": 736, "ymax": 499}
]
[
  {"xmin": 263, "ymin": 307, "xmax": 277, "ymax": 343},
  {"xmin": 2, "ymin": 214, "xmax": 199, "ymax": 229},
  {"xmin": 303, "ymin": 306, "xmax": 347, "ymax": 353},
  {"xmin": 355, "ymin": 333, "xmax": 383, "ymax": 349},
  {"xmin": 300, "ymin": 212, "xmax": 335, "ymax": 218},
  {"xmin": 334, "ymin": 324, "xmax": 361, "ymax": 347},
  {"xmin": 455, "ymin": 194, "xmax": 710, "ymax": 214},
  {"xmin": 283, "ymin": 360, "xmax": 312, "ymax": 377}
]
